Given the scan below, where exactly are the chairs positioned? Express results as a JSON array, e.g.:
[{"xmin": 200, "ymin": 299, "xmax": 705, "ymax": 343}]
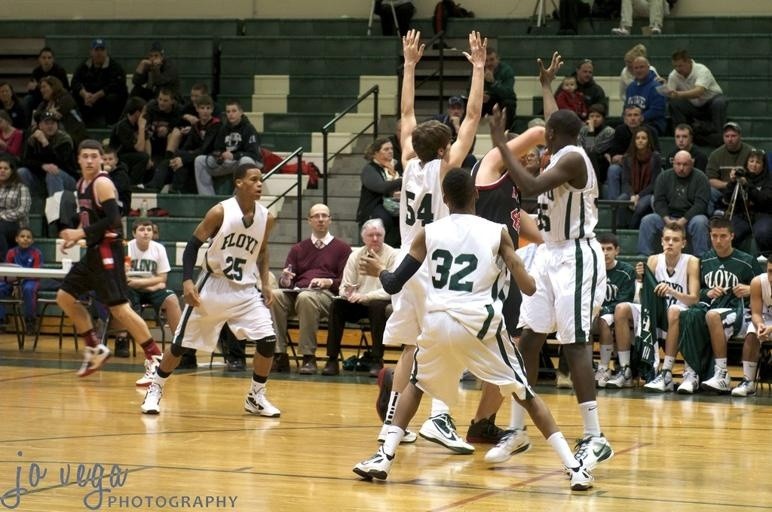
[{"xmin": 0, "ymin": 263, "xmax": 772, "ymax": 395}]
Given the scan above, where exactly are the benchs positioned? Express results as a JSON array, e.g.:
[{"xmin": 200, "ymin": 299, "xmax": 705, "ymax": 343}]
[{"xmin": 0, "ymin": 16, "xmax": 772, "ymax": 319}]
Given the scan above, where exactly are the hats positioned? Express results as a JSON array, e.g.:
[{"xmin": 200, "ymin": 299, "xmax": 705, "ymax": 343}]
[
  {"xmin": 90, "ymin": 39, "xmax": 105, "ymax": 48},
  {"xmin": 41, "ymin": 112, "xmax": 57, "ymax": 121},
  {"xmin": 722, "ymin": 122, "xmax": 742, "ymax": 131}
]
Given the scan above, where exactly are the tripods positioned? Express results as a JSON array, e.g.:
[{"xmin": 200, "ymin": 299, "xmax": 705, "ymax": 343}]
[{"xmin": 726, "ymin": 182, "xmax": 753, "ymax": 235}]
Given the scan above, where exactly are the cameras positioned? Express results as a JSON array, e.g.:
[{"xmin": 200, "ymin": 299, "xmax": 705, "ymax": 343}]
[{"xmin": 214, "ymin": 153, "xmax": 227, "ymax": 165}]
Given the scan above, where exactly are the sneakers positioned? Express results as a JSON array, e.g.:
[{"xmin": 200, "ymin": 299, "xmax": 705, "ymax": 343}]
[
  {"xmin": 554, "ymin": 370, "xmax": 574, "ymax": 387},
  {"xmin": 115, "ymin": 335, "xmax": 129, "ymax": 357},
  {"xmin": 594, "ymin": 364, "xmax": 757, "ymax": 396},
  {"xmin": 229, "ymin": 358, "xmax": 245, "ymax": 371},
  {"xmin": 376, "ymin": 365, "xmax": 530, "ymax": 464},
  {"xmin": 562, "ymin": 433, "xmax": 613, "ymax": 490},
  {"xmin": 353, "ymin": 445, "xmax": 395, "ymax": 480},
  {"xmin": 245, "ymin": 388, "xmax": 280, "ymax": 417},
  {"xmin": 76, "ymin": 344, "xmax": 111, "ymax": 377},
  {"xmin": 271, "ymin": 354, "xmax": 383, "ymax": 378},
  {"xmin": 612, "ymin": 25, "xmax": 662, "ymax": 35},
  {"xmin": 136, "ymin": 353, "xmax": 164, "ymax": 414}
]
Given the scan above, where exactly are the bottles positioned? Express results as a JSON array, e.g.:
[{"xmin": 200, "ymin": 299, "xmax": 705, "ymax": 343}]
[{"xmin": 140, "ymin": 200, "xmax": 148, "ymax": 219}]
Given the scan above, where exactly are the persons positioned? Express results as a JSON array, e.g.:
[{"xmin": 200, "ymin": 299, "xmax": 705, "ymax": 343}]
[
  {"xmin": 2, "ymin": 29, "xmax": 772, "ymax": 491},
  {"xmin": 611, "ymin": 0, "xmax": 677, "ymax": 36}
]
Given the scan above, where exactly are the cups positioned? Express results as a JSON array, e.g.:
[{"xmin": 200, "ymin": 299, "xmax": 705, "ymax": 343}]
[{"xmin": 62, "ymin": 259, "xmax": 72, "ymax": 270}]
